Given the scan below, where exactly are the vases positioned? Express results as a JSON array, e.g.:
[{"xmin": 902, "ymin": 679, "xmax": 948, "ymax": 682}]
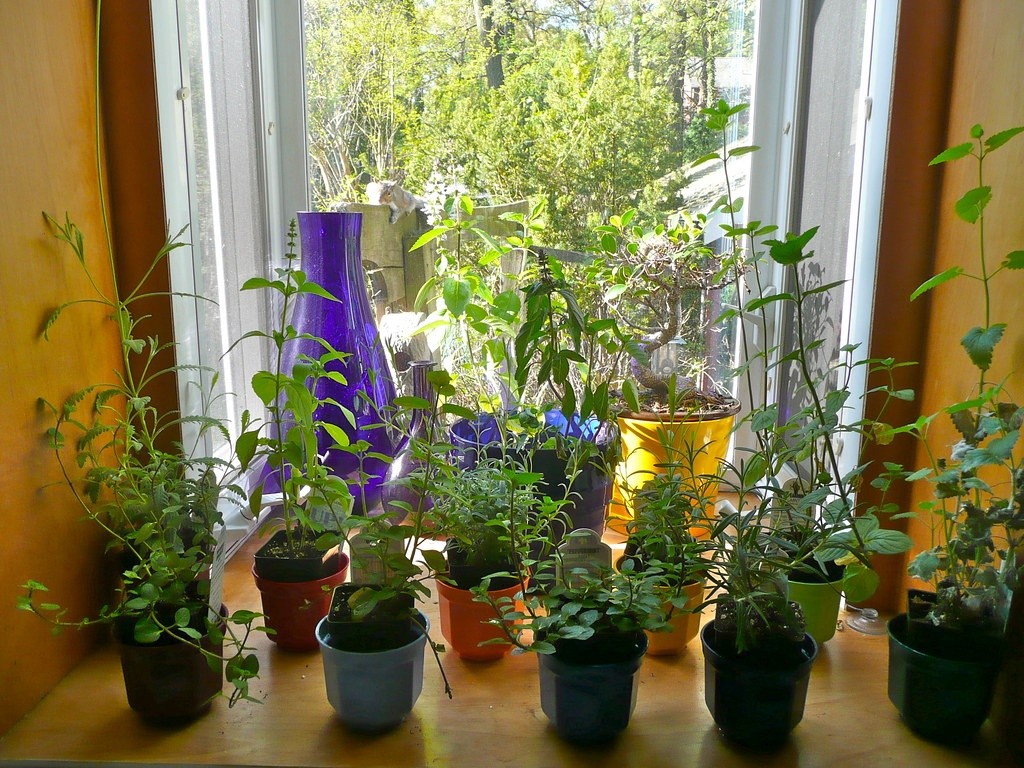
[
  {"xmin": 380, "ymin": 362, "xmax": 457, "ymax": 536},
  {"xmin": 278, "ymin": 209, "xmax": 406, "ymax": 519}
]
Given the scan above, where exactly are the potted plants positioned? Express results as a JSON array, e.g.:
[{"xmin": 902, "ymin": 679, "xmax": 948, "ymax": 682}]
[{"xmin": 13, "ymin": 95, "xmax": 1024, "ymax": 752}]
